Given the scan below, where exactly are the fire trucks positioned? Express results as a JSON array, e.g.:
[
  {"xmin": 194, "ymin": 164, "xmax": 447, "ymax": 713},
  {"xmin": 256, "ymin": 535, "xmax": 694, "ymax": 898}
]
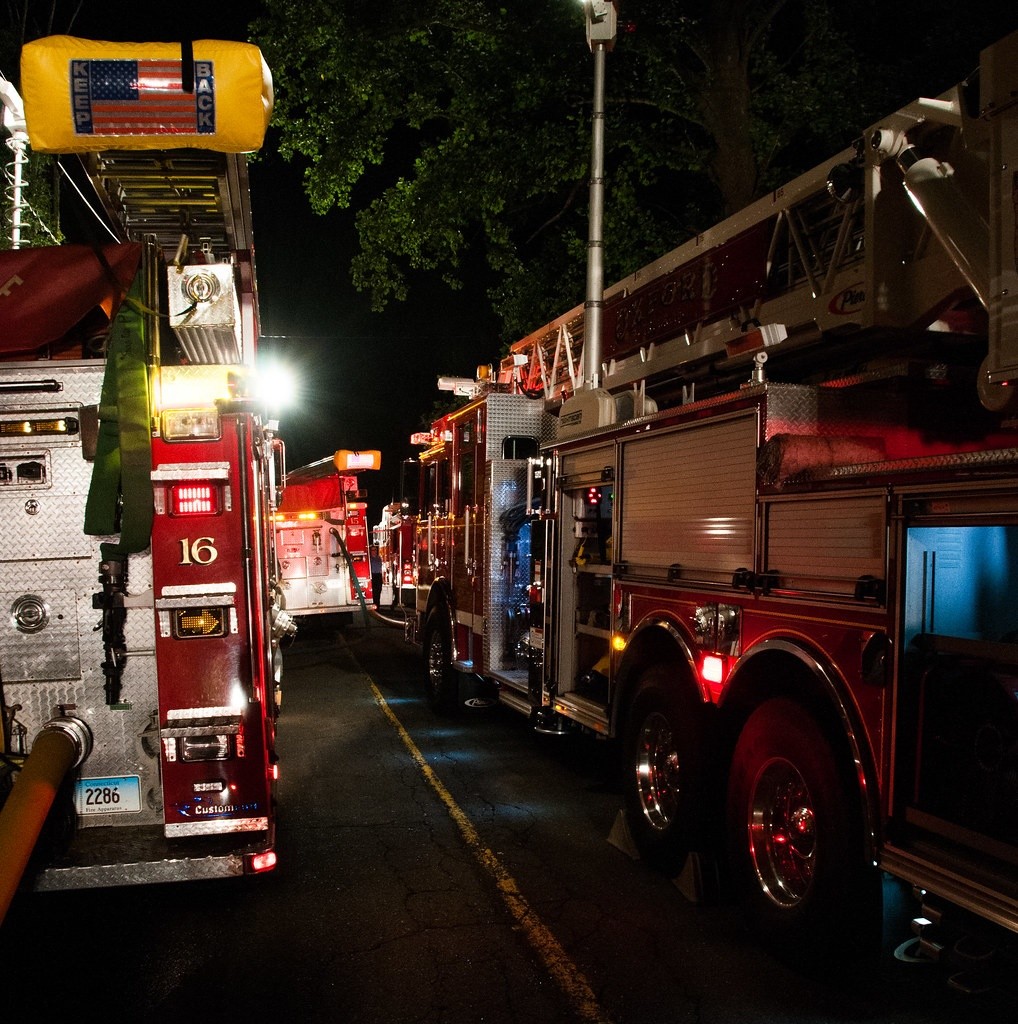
[
  {"xmin": 0, "ymin": 33, "xmax": 297, "ymax": 938},
  {"xmin": 268, "ymin": 451, "xmax": 385, "ymax": 629},
  {"xmin": 372, "ymin": 22, "xmax": 1018, "ymax": 958}
]
